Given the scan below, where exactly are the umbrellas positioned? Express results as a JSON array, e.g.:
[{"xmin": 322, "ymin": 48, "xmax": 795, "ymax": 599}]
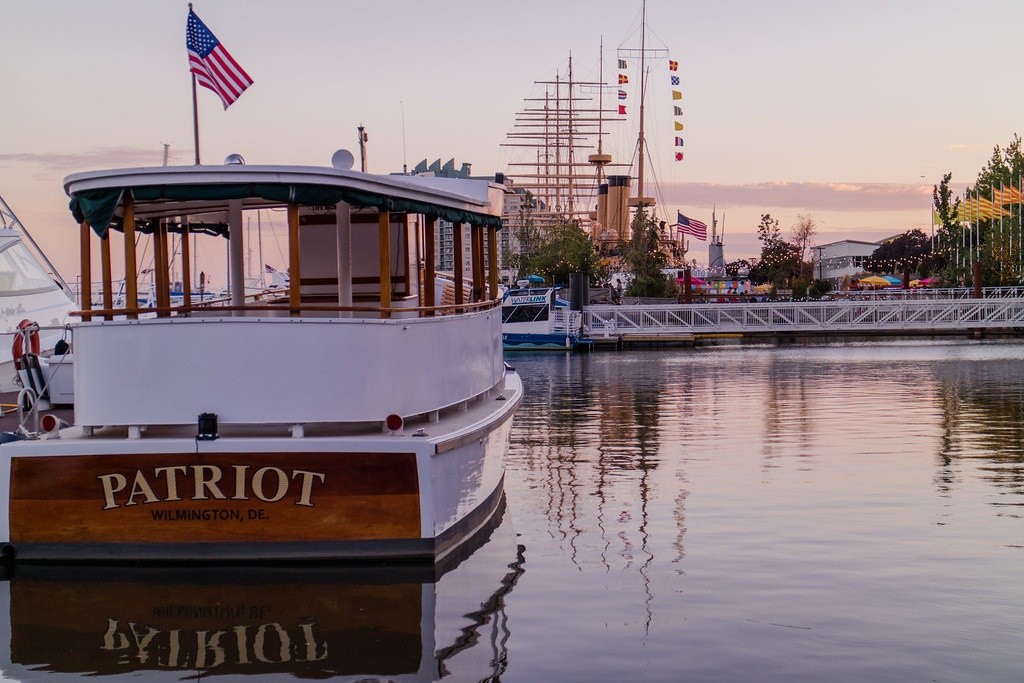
[
  {"xmin": 859, "ymin": 274, "xmax": 902, "ymax": 286},
  {"xmin": 675, "ymin": 275, "xmax": 706, "ymax": 285},
  {"xmin": 919, "ymin": 276, "xmax": 950, "ymax": 284}
]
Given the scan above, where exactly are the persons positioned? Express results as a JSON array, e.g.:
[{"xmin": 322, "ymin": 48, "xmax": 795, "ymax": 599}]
[
  {"xmin": 615, "ymin": 279, "xmax": 622, "ymax": 305},
  {"xmin": 727, "ymin": 287, "xmax": 767, "ymax": 303}
]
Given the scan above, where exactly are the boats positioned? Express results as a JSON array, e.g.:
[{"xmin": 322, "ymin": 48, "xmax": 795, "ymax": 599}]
[
  {"xmin": 0, "ymin": 3, "xmax": 526, "ymax": 583},
  {"xmin": 493, "ymin": 274, "xmax": 577, "ymax": 348}
]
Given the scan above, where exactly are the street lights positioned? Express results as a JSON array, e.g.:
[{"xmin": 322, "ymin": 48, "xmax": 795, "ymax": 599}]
[
  {"xmin": 817, "ymin": 247, "xmax": 826, "ymax": 281},
  {"xmin": 749, "ymin": 258, "xmax": 756, "ymax": 266}
]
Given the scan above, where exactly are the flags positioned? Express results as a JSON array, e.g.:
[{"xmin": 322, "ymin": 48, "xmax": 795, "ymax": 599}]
[
  {"xmin": 670, "ymin": 75, "xmax": 680, "ymax": 85},
  {"xmin": 676, "ymin": 213, "xmax": 708, "ymax": 242},
  {"xmin": 669, "ymin": 60, "xmax": 679, "ymax": 72},
  {"xmin": 674, "ymin": 152, "xmax": 685, "ymax": 162},
  {"xmin": 618, "ymin": 90, "xmax": 627, "ymax": 100},
  {"xmin": 185, "ymin": 7, "xmax": 254, "ymax": 110},
  {"xmin": 618, "ymin": 74, "xmax": 628, "ymax": 84},
  {"xmin": 674, "ymin": 121, "xmax": 683, "ymax": 131},
  {"xmin": 675, "ymin": 136, "xmax": 684, "ymax": 147},
  {"xmin": 673, "ymin": 106, "xmax": 683, "ymax": 115},
  {"xmin": 618, "ymin": 59, "xmax": 628, "ymax": 69},
  {"xmin": 619, "ymin": 105, "xmax": 627, "ymax": 114},
  {"xmin": 672, "ymin": 90, "xmax": 682, "ymax": 100},
  {"xmin": 923, "ymin": 177, "xmax": 1024, "ymax": 225}
]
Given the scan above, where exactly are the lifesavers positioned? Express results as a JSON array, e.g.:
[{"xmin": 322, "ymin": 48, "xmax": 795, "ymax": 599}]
[{"xmin": 12, "ymin": 319, "xmax": 40, "ymax": 370}]
